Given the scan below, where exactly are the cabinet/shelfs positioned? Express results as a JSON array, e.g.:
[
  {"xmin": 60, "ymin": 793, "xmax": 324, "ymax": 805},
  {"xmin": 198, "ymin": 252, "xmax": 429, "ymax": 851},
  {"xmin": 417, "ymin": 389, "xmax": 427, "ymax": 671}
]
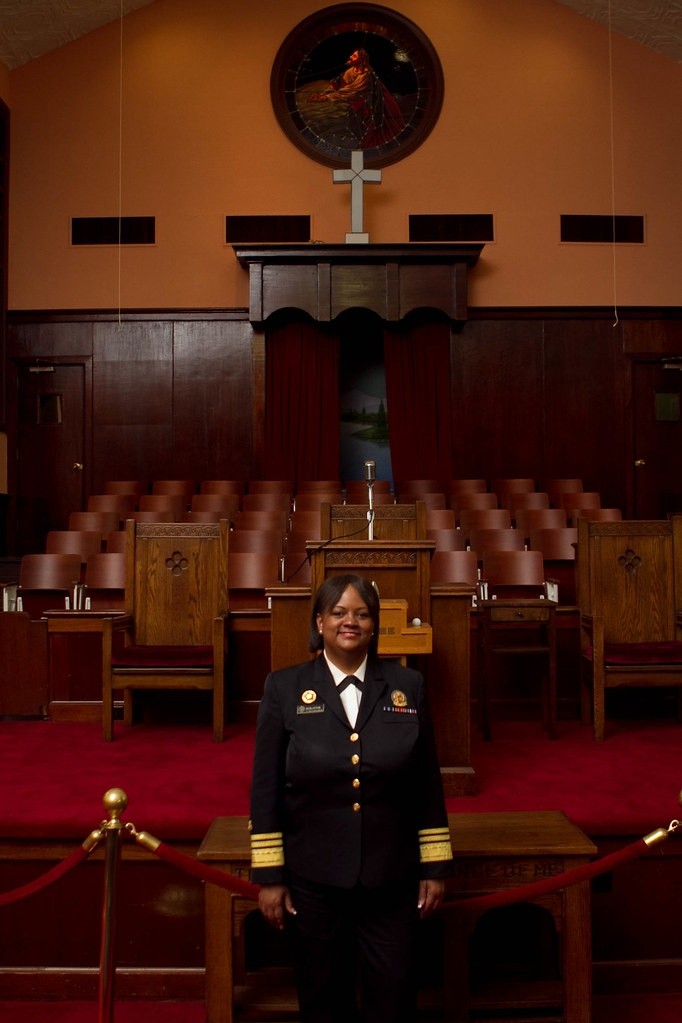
[{"xmin": 0, "ymin": 612, "xmax": 49, "ymax": 719}]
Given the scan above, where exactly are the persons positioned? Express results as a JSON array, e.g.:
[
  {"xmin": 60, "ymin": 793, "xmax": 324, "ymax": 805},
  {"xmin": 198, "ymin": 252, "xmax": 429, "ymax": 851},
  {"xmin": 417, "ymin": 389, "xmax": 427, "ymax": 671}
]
[{"xmin": 245, "ymin": 572, "xmax": 455, "ymax": 1022}]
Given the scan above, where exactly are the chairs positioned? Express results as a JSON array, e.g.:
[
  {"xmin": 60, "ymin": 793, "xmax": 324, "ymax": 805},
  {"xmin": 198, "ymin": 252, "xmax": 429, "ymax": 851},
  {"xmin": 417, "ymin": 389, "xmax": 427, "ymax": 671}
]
[
  {"xmin": 577, "ymin": 513, "xmax": 681, "ymax": 743},
  {"xmin": 19, "ymin": 476, "xmax": 622, "ymax": 752}
]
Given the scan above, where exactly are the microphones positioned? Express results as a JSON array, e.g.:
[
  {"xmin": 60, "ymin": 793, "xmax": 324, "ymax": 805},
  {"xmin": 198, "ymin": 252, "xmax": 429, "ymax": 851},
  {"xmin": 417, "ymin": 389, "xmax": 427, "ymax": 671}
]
[{"xmin": 364, "ymin": 461, "xmax": 377, "ymax": 515}]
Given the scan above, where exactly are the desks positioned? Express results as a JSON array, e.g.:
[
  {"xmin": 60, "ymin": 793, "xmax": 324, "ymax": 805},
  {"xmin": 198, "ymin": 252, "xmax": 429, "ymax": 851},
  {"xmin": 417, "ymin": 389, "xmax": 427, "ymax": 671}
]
[
  {"xmin": 198, "ymin": 809, "xmax": 594, "ymax": 1023},
  {"xmin": 266, "ymin": 541, "xmax": 478, "ymax": 799}
]
